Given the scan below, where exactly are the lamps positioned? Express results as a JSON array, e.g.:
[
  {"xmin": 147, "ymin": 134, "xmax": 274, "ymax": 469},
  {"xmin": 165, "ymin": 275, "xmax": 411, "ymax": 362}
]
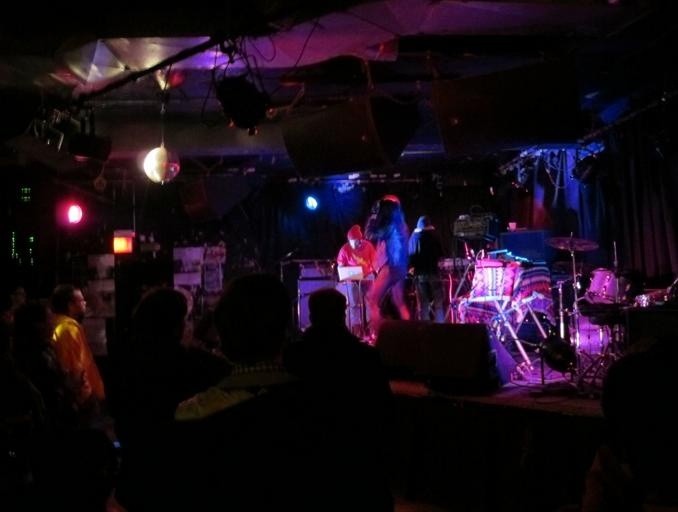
[{"xmin": 527, "ymin": 335, "xmax": 576, "ymax": 396}]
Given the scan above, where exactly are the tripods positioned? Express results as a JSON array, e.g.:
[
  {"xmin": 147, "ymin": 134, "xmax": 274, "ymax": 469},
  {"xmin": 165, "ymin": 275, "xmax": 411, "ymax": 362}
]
[{"xmin": 539, "ymin": 253, "xmax": 623, "ymax": 394}]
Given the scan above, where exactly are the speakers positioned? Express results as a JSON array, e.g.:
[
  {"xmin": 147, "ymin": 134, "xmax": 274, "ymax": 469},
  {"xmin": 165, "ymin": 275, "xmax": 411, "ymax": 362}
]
[
  {"xmin": 282, "ymin": 95, "xmax": 410, "ymax": 178},
  {"xmin": 433, "ymin": 51, "xmax": 573, "ymax": 151},
  {"xmin": 114, "ymin": 253, "xmax": 173, "ymax": 334},
  {"xmin": 375, "ymin": 319, "xmax": 495, "ymax": 392}
]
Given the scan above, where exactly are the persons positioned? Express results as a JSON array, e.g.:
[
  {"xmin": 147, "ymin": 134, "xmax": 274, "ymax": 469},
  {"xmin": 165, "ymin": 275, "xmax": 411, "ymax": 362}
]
[
  {"xmin": 376, "ymin": 215, "xmax": 426, "ymax": 271},
  {"xmin": 408, "ymin": 217, "xmax": 447, "ymax": 323},
  {"xmin": 337, "ymin": 224, "xmax": 382, "ymax": 339},
  {"xmin": 0, "ymin": 272, "xmax": 399, "ymax": 512},
  {"xmin": 363, "ymin": 195, "xmax": 412, "ymax": 346}
]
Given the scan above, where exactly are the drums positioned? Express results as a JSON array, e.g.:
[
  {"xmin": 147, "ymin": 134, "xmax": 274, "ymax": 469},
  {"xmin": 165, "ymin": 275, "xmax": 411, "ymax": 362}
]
[
  {"xmin": 636, "ymin": 287, "xmax": 670, "ymax": 308},
  {"xmin": 569, "ymin": 304, "xmax": 625, "ymax": 360},
  {"xmin": 585, "ymin": 267, "xmax": 633, "ymax": 305}
]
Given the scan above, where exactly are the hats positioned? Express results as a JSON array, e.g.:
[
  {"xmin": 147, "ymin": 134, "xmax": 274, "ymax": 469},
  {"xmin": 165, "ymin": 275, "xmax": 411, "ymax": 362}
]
[{"xmin": 348, "ymin": 224, "xmax": 363, "ymax": 241}]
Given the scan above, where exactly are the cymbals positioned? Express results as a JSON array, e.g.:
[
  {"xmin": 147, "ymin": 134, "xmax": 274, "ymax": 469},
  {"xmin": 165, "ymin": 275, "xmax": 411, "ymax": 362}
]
[
  {"xmin": 544, "ymin": 235, "xmax": 598, "ymax": 252},
  {"xmin": 554, "ymin": 258, "xmax": 598, "ymax": 272}
]
